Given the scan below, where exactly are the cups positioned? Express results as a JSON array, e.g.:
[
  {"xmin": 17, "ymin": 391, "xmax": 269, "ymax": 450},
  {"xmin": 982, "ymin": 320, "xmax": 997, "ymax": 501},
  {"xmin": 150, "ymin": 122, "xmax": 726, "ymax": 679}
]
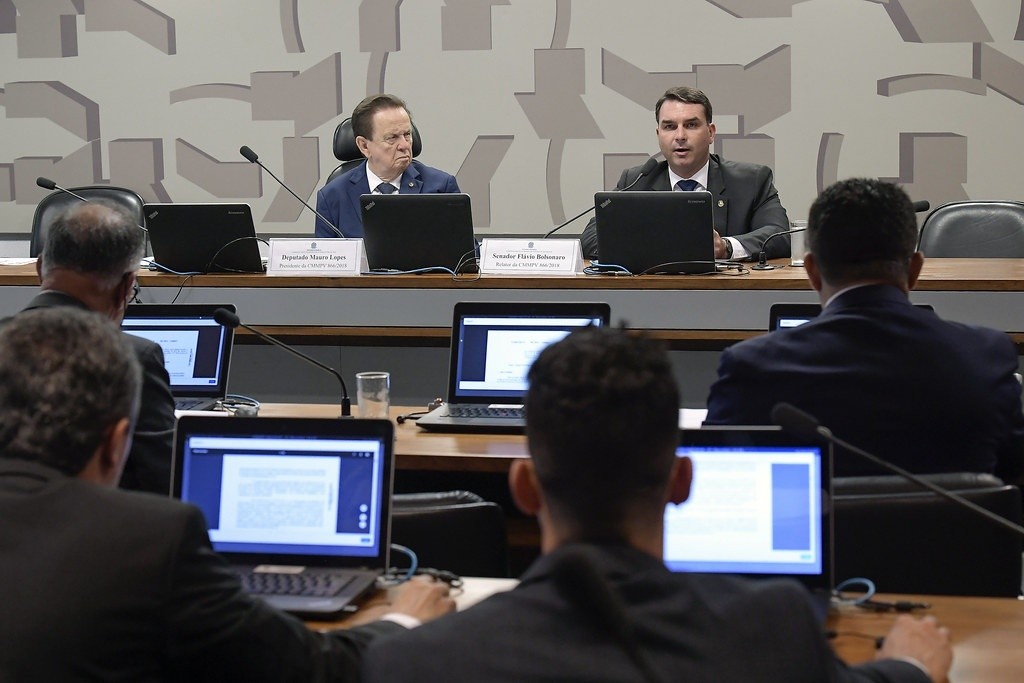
[
  {"xmin": 789, "ymin": 219, "xmax": 807, "ymax": 265},
  {"xmin": 355, "ymin": 372, "xmax": 391, "ymax": 419}
]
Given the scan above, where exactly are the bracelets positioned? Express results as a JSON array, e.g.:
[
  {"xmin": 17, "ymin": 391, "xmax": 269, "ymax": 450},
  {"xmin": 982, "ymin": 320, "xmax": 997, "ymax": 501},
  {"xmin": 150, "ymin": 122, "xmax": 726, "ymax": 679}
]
[{"xmin": 724, "ymin": 238, "xmax": 733, "ymax": 258}]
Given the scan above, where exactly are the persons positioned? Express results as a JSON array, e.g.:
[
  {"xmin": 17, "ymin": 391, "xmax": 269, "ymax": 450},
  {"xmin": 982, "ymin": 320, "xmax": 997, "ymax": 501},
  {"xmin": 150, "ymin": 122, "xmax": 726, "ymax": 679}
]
[
  {"xmin": 705, "ymin": 178, "xmax": 1024, "ymax": 483},
  {"xmin": 582, "ymin": 86, "xmax": 791, "ymax": 261},
  {"xmin": 0, "ymin": 197, "xmax": 176, "ymax": 494},
  {"xmin": 362, "ymin": 324, "xmax": 951, "ymax": 683},
  {"xmin": 0, "ymin": 305, "xmax": 457, "ymax": 683},
  {"xmin": 316, "ymin": 95, "xmax": 461, "ymax": 238}
]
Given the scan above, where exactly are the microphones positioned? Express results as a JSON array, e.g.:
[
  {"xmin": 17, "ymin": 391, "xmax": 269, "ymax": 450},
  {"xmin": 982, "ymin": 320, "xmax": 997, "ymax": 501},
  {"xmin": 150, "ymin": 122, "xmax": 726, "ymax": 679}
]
[
  {"xmin": 213, "ymin": 308, "xmax": 356, "ymax": 417},
  {"xmin": 547, "ymin": 158, "xmax": 658, "ymax": 239},
  {"xmin": 771, "ymin": 404, "xmax": 1024, "ymax": 539},
  {"xmin": 36, "ymin": 177, "xmax": 147, "ymax": 234},
  {"xmin": 240, "ymin": 146, "xmax": 345, "ymax": 238},
  {"xmin": 752, "ymin": 200, "xmax": 931, "ymax": 270}
]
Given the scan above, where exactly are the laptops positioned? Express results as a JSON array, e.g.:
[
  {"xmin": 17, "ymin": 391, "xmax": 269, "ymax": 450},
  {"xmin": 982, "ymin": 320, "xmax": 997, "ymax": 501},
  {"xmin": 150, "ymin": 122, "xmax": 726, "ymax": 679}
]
[
  {"xmin": 143, "ymin": 202, "xmax": 269, "ymax": 274},
  {"xmin": 660, "ymin": 426, "xmax": 833, "ymax": 634},
  {"xmin": 360, "ymin": 194, "xmax": 482, "ymax": 273},
  {"xmin": 120, "ymin": 303, "xmax": 237, "ymax": 410},
  {"xmin": 768, "ymin": 303, "xmax": 934, "ymax": 335},
  {"xmin": 170, "ymin": 415, "xmax": 395, "ymax": 620},
  {"xmin": 415, "ymin": 302, "xmax": 611, "ymax": 435},
  {"xmin": 594, "ymin": 190, "xmax": 731, "ymax": 274}
]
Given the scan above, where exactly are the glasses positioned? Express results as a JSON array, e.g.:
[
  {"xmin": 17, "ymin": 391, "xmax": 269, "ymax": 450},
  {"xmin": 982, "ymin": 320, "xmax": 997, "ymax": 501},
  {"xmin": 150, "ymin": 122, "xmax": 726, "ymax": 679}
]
[{"xmin": 125, "ymin": 272, "xmax": 140, "ymax": 303}]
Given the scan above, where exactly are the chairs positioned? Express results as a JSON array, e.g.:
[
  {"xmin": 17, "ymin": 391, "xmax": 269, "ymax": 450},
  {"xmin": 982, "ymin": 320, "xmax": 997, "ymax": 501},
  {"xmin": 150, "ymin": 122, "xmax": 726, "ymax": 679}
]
[
  {"xmin": 390, "ymin": 490, "xmax": 513, "ymax": 580},
  {"xmin": 30, "ymin": 187, "xmax": 148, "ymax": 260},
  {"xmin": 918, "ymin": 200, "xmax": 1024, "ymax": 259},
  {"xmin": 324, "ymin": 117, "xmax": 422, "ymax": 182}
]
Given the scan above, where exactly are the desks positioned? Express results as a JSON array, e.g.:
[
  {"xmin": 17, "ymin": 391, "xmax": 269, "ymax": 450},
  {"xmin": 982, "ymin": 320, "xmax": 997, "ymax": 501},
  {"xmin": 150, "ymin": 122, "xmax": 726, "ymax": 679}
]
[
  {"xmin": 303, "ymin": 575, "xmax": 1024, "ymax": 683},
  {"xmin": 174, "ymin": 402, "xmax": 707, "ymax": 552}
]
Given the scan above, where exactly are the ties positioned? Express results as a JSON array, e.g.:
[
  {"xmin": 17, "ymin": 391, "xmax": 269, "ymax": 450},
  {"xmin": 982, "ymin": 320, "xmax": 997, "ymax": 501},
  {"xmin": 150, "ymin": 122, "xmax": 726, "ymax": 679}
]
[
  {"xmin": 377, "ymin": 183, "xmax": 396, "ymax": 195},
  {"xmin": 677, "ymin": 179, "xmax": 699, "ymax": 192}
]
